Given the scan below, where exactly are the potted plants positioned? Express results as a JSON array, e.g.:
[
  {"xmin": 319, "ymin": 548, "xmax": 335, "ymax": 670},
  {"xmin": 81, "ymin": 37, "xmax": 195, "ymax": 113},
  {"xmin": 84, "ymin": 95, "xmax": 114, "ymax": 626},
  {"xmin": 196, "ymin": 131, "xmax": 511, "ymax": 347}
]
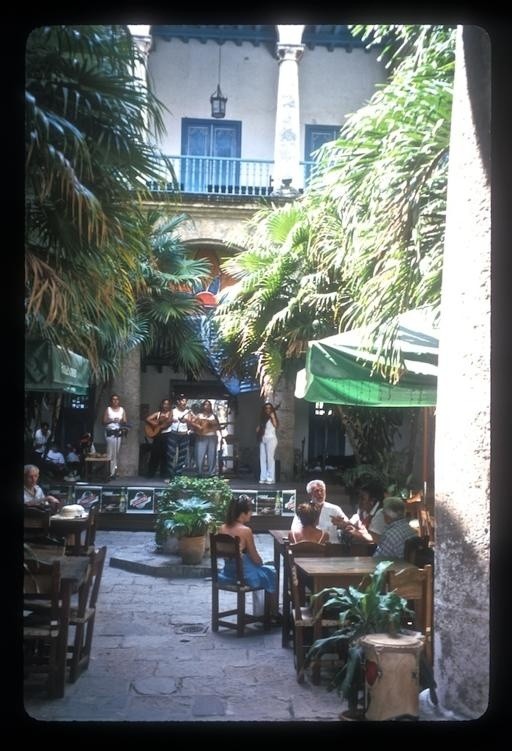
[
  {"xmin": 303, "ymin": 560, "xmax": 408, "ymax": 720},
  {"xmin": 153, "ymin": 473, "xmax": 235, "ymax": 556},
  {"xmin": 164, "ymin": 496, "xmax": 218, "ymax": 565}
]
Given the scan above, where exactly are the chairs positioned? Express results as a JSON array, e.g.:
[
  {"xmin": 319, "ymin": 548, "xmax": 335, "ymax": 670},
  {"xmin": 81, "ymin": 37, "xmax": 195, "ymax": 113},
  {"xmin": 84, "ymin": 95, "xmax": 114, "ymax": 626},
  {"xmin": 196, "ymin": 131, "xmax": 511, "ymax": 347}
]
[
  {"xmin": 273, "ymin": 521, "xmax": 431, "ymax": 686},
  {"xmin": 21, "ymin": 492, "xmax": 109, "ymax": 699},
  {"xmin": 210, "ymin": 532, "xmax": 270, "ymax": 640},
  {"xmin": 79, "ymin": 434, "xmax": 111, "ymax": 484},
  {"xmin": 217, "ymin": 432, "xmax": 240, "ymax": 478}
]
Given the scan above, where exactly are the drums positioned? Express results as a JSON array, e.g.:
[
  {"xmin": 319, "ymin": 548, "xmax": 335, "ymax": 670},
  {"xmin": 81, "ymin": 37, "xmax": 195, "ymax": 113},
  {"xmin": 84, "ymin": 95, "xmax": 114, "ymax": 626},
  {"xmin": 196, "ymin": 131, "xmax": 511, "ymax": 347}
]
[{"xmin": 104, "ymin": 420, "xmax": 121, "ymax": 436}]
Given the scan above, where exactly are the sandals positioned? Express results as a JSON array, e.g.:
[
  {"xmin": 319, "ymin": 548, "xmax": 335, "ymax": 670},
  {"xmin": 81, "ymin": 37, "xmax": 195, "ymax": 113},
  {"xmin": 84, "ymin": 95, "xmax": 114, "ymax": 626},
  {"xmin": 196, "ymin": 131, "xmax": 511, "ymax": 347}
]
[{"xmin": 270, "ymin": 612, "xmax": 283, "ymax": 627}]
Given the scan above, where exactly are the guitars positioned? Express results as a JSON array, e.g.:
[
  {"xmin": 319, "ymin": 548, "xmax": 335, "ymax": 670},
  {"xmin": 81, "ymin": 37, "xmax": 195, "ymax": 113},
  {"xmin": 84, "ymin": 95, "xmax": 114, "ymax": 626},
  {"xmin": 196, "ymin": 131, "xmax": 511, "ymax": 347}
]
[
  {"xmin": 193, "ymin": 418, "xmax": 241, "ymax": 436},
  {"xmin": 258, "ymin": 402, "xmax": 281, "ymax": 439},
  {"xmin": 146, "ymin": 416, "xmax": 179, "ymax": 438}
]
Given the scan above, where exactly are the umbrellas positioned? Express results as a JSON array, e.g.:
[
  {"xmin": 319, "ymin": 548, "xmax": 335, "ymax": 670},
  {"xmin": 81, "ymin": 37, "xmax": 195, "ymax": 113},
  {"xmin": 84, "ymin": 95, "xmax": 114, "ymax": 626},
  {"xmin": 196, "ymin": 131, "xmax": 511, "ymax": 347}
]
[{"xmin": 293, "ymin": 306, "xmax": 438, "ymax": 502}]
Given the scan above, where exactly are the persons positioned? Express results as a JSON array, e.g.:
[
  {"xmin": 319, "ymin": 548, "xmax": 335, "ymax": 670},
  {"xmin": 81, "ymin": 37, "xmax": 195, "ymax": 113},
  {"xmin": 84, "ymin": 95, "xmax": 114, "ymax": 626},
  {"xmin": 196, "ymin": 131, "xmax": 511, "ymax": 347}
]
[
  {"xmin": 23, "ymin": 465, "xmax": 60, "ymax": 507},
  {"xmin": 145, "ymin": 394, "xmax": 227, "ymax": 484},
  {"xmin": 288, "ymin": 474, "xmax": 418, "ymax": 559},
  {"xmin": 256, "ymin": 403, "xmax": 278, "ymax": 485},
  {"xmin": 219, "ymin": 495, "xmax": 282, "ymax": 628},
  {"xmin": 33, "ymin": 422, "xmax": 54, "ymax": 453},
  {"xmin": 101, "ymin": 395, "xmax": 128, "ymax": 477}
]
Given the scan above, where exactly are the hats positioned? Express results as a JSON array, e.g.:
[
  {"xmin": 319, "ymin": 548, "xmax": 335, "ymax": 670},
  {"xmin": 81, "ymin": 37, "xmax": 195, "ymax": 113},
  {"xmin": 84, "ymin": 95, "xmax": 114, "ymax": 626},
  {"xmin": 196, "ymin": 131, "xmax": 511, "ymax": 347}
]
[{"xmin": 50, "ymin": 504, "xmax": 89, "ymax": 520}]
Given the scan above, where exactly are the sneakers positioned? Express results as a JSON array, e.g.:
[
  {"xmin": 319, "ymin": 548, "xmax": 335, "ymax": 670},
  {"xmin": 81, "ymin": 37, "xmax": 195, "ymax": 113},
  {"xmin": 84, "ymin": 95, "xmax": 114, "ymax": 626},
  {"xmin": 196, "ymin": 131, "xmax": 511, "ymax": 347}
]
[{"xmin": 259, "ymin": 479, "xmax": 276, "ymax": 485}]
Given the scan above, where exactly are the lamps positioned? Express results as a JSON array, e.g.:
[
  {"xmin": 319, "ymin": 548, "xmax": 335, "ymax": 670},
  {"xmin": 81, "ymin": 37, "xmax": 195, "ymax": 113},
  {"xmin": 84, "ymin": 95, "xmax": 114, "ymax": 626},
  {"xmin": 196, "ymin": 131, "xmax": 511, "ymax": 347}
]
[{"xmin": 209, "ymin": 39, "xmax": 229, "ymax": 119}]
